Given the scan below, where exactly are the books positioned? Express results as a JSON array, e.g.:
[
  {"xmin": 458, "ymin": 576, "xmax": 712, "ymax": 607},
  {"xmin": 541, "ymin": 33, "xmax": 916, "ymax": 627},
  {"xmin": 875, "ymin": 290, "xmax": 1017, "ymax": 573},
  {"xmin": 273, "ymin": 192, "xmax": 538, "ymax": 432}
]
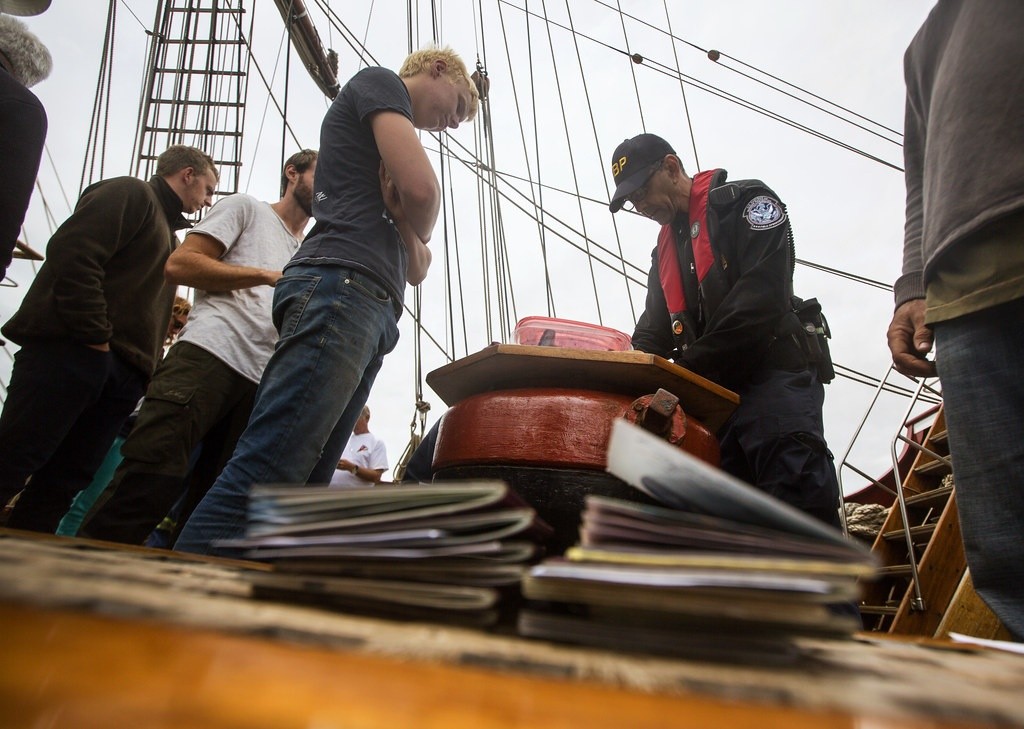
[
  {"xmin": 223, "ymin": 478, "xmax": 536, "ymax": 609},
  {"xmin": 516, "ymin": 406, "xmax": 874, "ymax": 660}
]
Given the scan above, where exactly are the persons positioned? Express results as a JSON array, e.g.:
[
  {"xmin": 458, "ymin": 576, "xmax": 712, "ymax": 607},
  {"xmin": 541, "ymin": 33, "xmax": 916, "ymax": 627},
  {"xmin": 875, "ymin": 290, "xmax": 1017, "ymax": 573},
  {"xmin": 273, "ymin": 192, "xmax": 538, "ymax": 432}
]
[
  {"xmin": 327, "ymin": 405, "xmax": 389, "ymax": 488},
  {"xmin": 0, "ymin": 146, "xmax": 221, "ymax": 534},
  {"xmin": 887, "ymin": 0, "xmax": 1024, "ymax": 642},
  {"xmin": 609, "ymin": 134, "xmax": 844, "ymax": 529},
  {"xmin": 0, "ymin": 8, "xmax": 53, "ymax": 282},
  {"xmin": 172, "ymin": 49, "xmax": 476, "ymax": 560},
  {"xmin": 74, "ymin": 146, "xmax": 319, "ymax": 546}
]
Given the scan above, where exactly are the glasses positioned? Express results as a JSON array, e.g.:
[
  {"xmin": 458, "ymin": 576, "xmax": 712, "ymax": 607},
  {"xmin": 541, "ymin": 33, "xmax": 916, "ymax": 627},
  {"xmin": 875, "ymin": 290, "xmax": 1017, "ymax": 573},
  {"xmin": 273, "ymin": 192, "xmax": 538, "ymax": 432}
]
[{"xmin": 619, "ymin": 162, "xmax": 663, "ymax": 211}]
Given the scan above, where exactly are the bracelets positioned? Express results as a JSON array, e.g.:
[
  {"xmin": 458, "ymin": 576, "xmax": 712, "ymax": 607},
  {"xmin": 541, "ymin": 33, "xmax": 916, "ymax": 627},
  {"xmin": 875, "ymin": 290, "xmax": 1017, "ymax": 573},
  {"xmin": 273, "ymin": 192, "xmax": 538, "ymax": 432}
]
[{"xmin": 350, "ymin": 465, "xmax": 359, "ymax": 476}]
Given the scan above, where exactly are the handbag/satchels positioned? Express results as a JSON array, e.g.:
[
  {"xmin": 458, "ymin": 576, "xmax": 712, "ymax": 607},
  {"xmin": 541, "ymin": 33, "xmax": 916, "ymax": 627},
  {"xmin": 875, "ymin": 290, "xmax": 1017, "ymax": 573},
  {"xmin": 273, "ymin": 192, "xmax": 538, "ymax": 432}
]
[{"xmin": 775, "ymin": 297, "xmax": 836, "ymax": 385}]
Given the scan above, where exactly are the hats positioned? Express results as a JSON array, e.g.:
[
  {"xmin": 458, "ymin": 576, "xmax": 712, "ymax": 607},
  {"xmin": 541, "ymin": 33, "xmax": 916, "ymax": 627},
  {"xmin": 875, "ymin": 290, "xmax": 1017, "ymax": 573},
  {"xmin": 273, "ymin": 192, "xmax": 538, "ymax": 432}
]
[{"xmin": 608, "ymin": 133, "xmax": 677, "ymax": 214}]
